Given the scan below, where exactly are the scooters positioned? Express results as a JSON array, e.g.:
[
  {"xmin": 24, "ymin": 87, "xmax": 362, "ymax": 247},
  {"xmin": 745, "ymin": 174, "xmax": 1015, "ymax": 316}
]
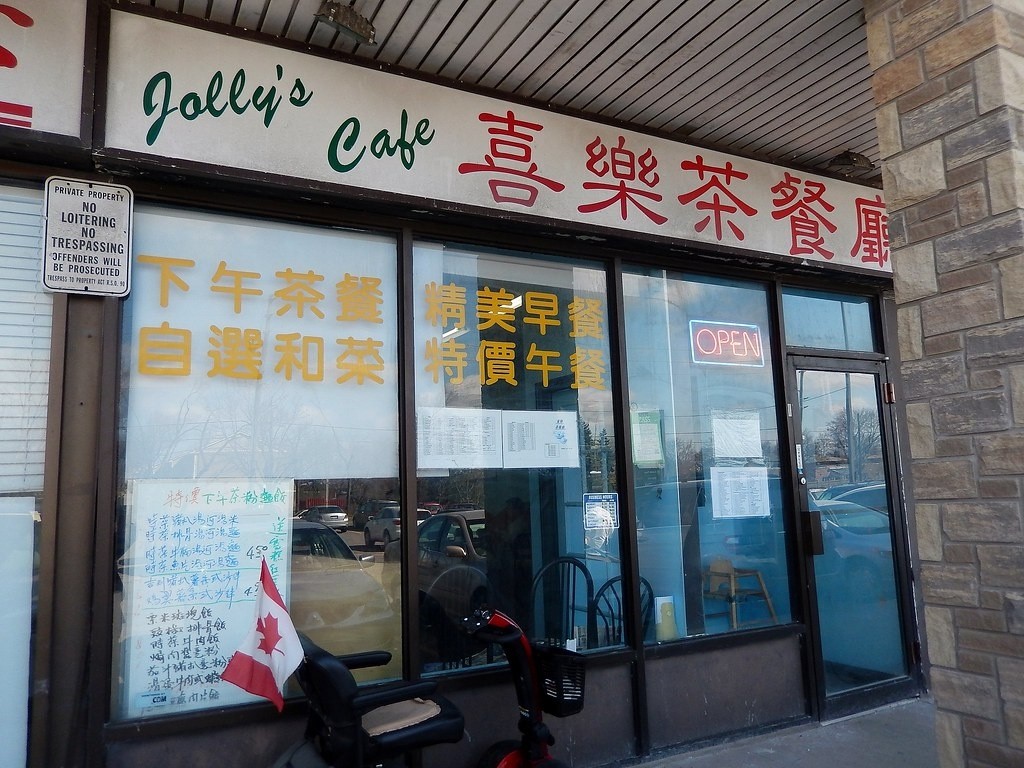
[{"xmin": 268, "ymin": 604, "xmax": 587, "ymax": 768}]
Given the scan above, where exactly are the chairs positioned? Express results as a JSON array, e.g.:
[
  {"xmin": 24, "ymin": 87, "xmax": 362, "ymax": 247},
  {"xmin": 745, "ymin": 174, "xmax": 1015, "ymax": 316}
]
[
  {"xmin": 593, "ymin": 574, "xmax": 654, "ymax": 647},
  {"xmin": 455, "ymin": 527, "xmax": 474, "ymax": 549},
  {"xmin": 273, "ymin": 629, "xmax": 464, "ymax": 768}
]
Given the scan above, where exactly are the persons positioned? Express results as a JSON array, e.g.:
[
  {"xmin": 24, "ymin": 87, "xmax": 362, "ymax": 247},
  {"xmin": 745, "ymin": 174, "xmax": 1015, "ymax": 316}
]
[{"xmin": 492, "ymin": 497, "xmax": 531, "ymax": 579}]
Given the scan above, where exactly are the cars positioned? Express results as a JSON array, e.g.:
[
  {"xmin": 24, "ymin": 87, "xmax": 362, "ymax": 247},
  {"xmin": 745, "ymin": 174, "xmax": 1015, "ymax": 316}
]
[
  {"xmin": 634, "ymin": 456, "xmax": 905, "ymax": 677},
  {"xmin": 293, "ymin": 506, "xmax": 349, "ymax": 533},
  {"xmin": 290, "ymin": 522, "xmax": 403, "ymax": 690},
  {"xmin": 364, "ymin": 507, "xmax": 432, "ymax": 548},
  {"xmin": 381, "ymin": 510, "xmax": 503, "ymax": 616},
  {"xmin": 352, "ymin": 499, "xmax": 481, "ymax": 529}
]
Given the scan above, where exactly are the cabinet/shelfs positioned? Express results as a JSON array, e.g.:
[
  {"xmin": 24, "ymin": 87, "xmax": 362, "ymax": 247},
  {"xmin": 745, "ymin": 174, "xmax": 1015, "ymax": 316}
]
[{"xmin": 702, "ymin": 559, "xmax": 778, "ymax": 631}]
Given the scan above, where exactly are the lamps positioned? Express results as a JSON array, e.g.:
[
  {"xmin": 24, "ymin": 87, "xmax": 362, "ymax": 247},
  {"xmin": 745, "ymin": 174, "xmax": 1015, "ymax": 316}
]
[
  {"xmin": 827, "ymin": 150, "xmax": 875, "ymax": 171},
  {"xmin": 314, "ymin": 2, "xmax": 379, "ymax": 47}
]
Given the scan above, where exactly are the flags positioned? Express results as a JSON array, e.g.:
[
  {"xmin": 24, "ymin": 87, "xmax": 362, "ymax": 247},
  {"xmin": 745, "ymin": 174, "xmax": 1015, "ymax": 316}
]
[{"xmin": 220, "ymin": 556, "xmax": 304, "ymax": 713}]
